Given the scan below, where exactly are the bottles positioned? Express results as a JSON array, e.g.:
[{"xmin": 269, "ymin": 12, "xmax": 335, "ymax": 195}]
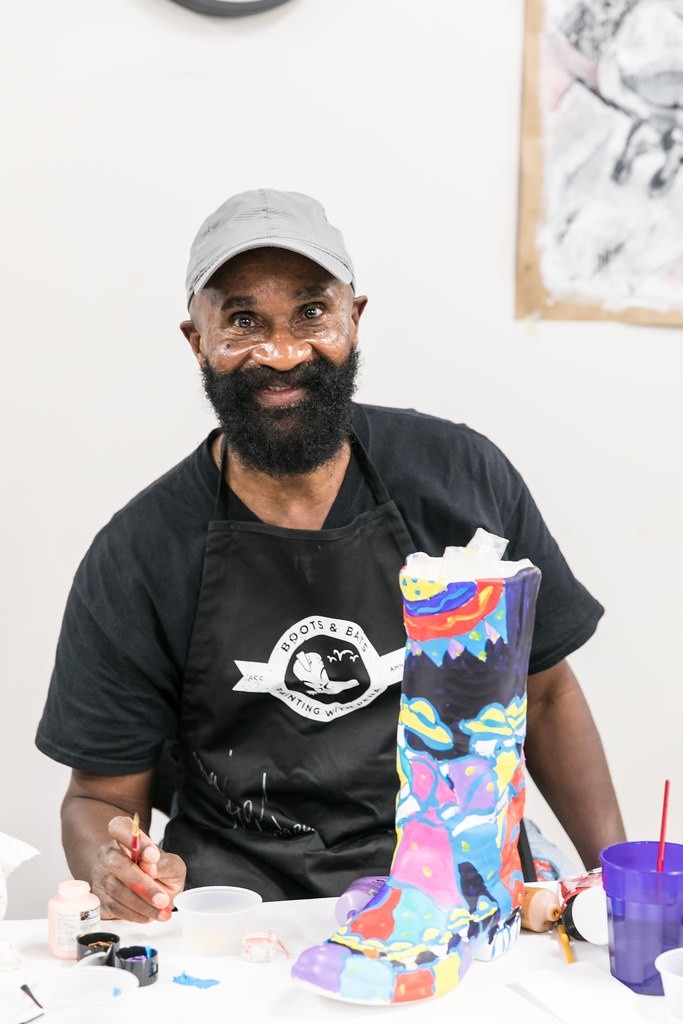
[{"xmin": 48, "ymin": 880, "xmax": 102, "ymax": 960}]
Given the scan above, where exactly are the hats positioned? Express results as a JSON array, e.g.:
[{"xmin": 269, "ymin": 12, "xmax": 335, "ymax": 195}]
[{"xmin": 187, "ymin": 192, "xmax": 355, "ymax": 313}]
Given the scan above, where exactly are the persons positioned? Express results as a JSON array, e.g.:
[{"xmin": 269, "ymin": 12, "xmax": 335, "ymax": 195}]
[{"xmin": 60, "ymin": 188, "xmax": 629, "ymax": 924}]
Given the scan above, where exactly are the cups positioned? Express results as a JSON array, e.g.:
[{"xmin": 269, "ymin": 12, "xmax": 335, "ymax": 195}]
[
  {"xmin": 655, "ymin": 947, "xmax": 683, "ymax": 1024},
  {"xmin": 76, "ymin": 930, "xmax": 121, "ymax": 966},
  {"xmin": 561, "ymin": 887, "xmax": 608, "ymax": 946},
  {"xmin": 115, "ymin": 946, "xmax": 159, "ymax": 986},
  {"xmin": 598, "ymin": 841, "xmax": 683, "ymax": 991}
]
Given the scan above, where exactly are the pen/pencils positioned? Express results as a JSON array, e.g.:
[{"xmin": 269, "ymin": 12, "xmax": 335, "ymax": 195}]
[
  {"xmin": 130, "ymin": 809, "xmax": 141, "ymax": 863},
  {"xmin": 557, "ymin": 917, "xmax": 575, "ymax": 964}
]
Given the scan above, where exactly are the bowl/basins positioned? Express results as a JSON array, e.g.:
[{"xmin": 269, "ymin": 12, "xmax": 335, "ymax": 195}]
[{"xmin": 173, "ymin": 886, "xmax": 262, "ymax": 955}]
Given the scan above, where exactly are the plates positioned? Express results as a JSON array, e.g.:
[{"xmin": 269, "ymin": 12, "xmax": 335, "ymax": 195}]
[{"xmin": 50, "ymin": 968, "xmax": 139, "ymax": 1002}]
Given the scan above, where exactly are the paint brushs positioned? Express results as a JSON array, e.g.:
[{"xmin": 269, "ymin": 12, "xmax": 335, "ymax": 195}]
[{"xmin": 656, "ymin": 779, "xmax": 671, "ymax": 873}]
